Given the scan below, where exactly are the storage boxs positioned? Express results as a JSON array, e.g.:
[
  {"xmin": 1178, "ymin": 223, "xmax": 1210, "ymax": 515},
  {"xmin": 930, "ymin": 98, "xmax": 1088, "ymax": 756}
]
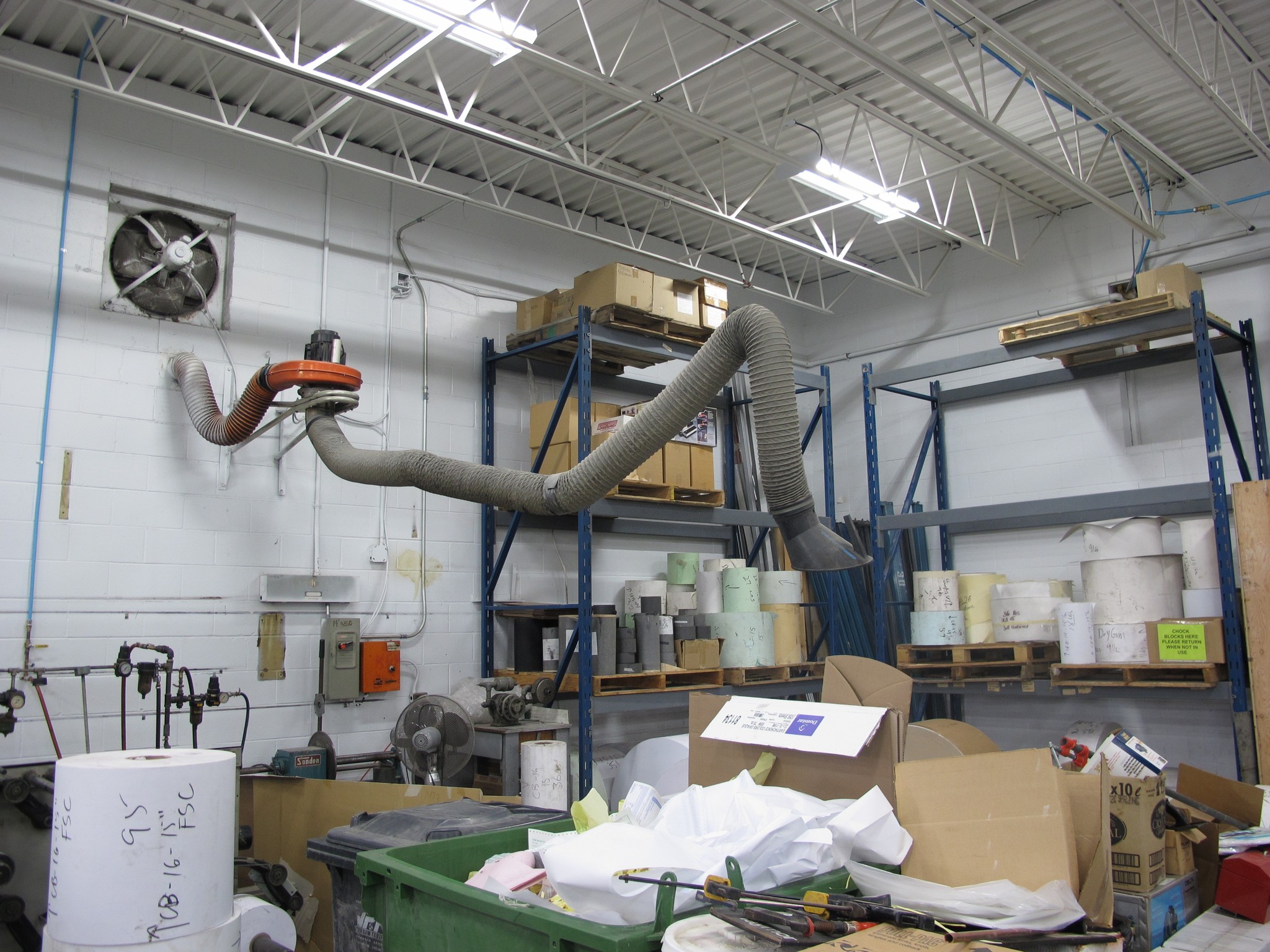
[
  {"xmin": 674, "ymin": 637, "xmax": 726, "ymax": 670},
  {"xmin": 1144, "ymin": 616, "xmax": 1227, "ymax": 665},
  {"xmin": 237, "ymin": 690, "xmax": 1270, "ymax": 952},
  {"xmin": 514, "ymin": 261, "xmax": 730, "ymax": 341},
  {"xmin": 528, "ymin": 395, "xmax": 716, "ymax": 491},
  {"xmin": 1134, "ymin": 263, "xmax": 1202, "ymax": 307}
]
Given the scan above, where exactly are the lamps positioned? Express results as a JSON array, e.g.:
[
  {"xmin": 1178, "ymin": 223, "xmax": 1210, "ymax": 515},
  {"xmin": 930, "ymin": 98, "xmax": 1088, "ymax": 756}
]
[
  {"xmin": 355, "ymin": 0, "xmax": 538, "ymax": 67},
  {"xmin": 786, "ymin": 130, "xmax": 921, "ymax": 226}
]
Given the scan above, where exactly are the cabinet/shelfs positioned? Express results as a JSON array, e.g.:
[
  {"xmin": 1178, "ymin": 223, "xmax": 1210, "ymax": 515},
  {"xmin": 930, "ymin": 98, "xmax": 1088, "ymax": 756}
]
[
  {"xmin": 476, "ymin": 305, "xmax": 839, "ymax": 798},
  {"xmin": 862, "ymin": 290, "xmax": 1270, "ymax": 784}
]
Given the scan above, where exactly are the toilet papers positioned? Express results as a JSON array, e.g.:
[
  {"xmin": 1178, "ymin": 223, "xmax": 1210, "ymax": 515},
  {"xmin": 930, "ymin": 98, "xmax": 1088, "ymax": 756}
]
[
  {"xmin": 624, "ymin": 551, "xmax": 804, "ymax": 668},
  {"xmin": 521, "ymin": 740, "xmax": 570, "ymax": 812},
  {"xmin": 43, "ymin": 748, "xmax": 241, "ymax": 952},
  {"xmin": 908, "ymin": 516, "xmax": 1223, "ymax": 665},
  {"xmin": 234, "ymin": 893, "xmax": 296, "ymax": 952}
]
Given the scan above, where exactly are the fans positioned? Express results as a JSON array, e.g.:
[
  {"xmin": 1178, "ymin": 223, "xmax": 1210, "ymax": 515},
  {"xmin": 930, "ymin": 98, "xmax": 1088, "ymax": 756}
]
[{"xmin": 394, "ymin": 694, "xmax": 476, "ymax": 786}]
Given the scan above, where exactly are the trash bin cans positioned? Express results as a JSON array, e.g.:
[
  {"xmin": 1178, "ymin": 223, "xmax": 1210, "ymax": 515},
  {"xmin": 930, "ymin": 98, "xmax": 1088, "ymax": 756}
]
[
  {"xmin": 305, "ymin": 796, "xmax": 572, "ymax": 952},
  {"xmin": 355, "ymin": 810, "xmax": 901, "ymax": 952},
  {"xmin": 662, "ymin": 913, "xmax": 1134, "ymax": 952}
]
